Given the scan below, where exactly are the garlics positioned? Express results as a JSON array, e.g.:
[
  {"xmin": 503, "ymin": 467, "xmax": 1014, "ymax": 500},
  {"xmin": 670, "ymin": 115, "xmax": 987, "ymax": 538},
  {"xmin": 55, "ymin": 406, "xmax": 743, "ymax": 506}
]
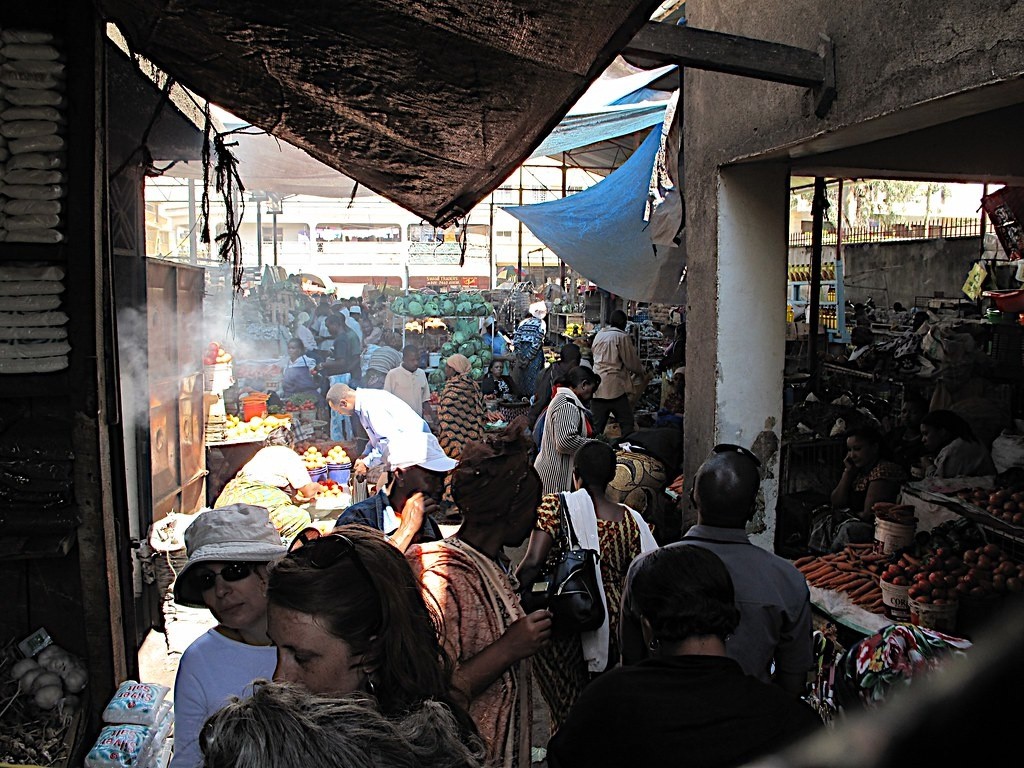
[{"xmin": 11, "ymin": 646, "xmax": 87, "ymax": 707}]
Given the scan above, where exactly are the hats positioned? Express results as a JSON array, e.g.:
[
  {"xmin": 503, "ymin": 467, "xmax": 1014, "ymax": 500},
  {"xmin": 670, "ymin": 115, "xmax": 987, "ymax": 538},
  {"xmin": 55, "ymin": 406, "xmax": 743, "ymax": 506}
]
[
  {"xmin": 296, "ymin": 312, "xmax": 310, "ymax": 325},
  {"xmin": 381, "ymin": 432, "xmax": 462, "ymax": 473},
  {"xmin": 349, "ymin": 306, "xmax": 362, "ymax": 314},
  {"xmin": 171, "ymin": 502, "xmax": 287, "ymax": 609},
  {"xmin": 329, "ymin": 299, "xmax": 342, "ymax": 306},
  {"xmin": 529, "ymin": 301, "xmax": 547, "ymax": 320},
  {"xmin": 483, "ymin": 316, "xmax": 497, "ymax": 328}
]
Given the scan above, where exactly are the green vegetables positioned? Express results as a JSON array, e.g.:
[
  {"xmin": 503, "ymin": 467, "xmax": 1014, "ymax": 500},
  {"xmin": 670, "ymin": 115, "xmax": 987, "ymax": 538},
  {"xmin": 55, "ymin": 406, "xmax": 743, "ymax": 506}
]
[
  {"xmin": 287, "ymin": 393, "xmax": 318, "ymax": 406},
  {"xmin": 268, "ymin": 403, "xmax": 286, "ymax": 411}
]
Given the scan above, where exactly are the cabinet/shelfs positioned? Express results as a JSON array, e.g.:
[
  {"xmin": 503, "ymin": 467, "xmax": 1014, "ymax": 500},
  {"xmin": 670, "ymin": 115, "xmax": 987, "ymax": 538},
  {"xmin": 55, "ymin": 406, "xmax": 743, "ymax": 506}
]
[{"xmin": 787, "ymin": 280, "xmax": 838, "ymax": 342}]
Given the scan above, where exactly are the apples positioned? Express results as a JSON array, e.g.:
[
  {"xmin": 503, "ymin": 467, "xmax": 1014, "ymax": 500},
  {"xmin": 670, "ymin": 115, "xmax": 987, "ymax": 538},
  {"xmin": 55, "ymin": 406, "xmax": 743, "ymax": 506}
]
[
  {"xmin": 204, "ymin": 341, "xmax": 232, "ymax": 364},
  {"xmin": 296, "ymin": 441, "xmax": 350, "ymax": 497},
  {"xmin": 225, "ymin": 414, "xmax": 279, "ymax": 437}
]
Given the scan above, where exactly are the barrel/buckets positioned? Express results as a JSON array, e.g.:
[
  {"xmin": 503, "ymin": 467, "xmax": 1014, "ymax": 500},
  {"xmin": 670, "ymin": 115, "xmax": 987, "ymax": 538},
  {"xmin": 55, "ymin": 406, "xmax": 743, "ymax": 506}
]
[
  {"xmin": 300, "ymin": 409, "xmax": 317, "ymax": 420},
  {"xmin": 288, "ymin": 412, "xmax": 300, "ymax": 418},
  {"xmin": 204, "ymin": 362, "xmax": 233, "ymax": 393},
  {"xmin": 307, "ymin": 466, "xmax": 328, "ymax": 482},
  {"xmin": 328, "ymin": 462, "xmax": 352, "ymax": 485},
  {"xmin": 874, "ymin": 517, "xmax": 917, "ymax": 556},
  {"xmin": 907, "ymin": 596, "xmax": 952, "ymax": 631},
  {"xmin": 879, "ymin": 576, "xmax": 912, "ymax": 622}
]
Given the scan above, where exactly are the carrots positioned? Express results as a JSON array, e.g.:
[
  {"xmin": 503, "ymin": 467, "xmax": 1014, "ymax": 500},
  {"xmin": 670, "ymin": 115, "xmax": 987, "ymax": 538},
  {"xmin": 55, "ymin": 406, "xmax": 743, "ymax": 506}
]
[
  {"xmin": 794, "ymin": 544, "xmax": 922, "ymax": 614},
  {"xmin": 871, "ymin": 502, "xmax": 920, "ymax": 526},
  {"xmin": 487, "ymin": 409, "xmax": 506, "ymax": 421},
  {"xmin": 668, "ymin": 476, "xmax": 683, "ymax": 494}
]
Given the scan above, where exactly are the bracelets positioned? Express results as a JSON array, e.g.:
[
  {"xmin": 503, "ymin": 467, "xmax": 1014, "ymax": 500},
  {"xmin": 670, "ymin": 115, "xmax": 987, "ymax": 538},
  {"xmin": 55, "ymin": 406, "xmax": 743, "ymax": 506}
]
[{"xmin": 844, "ymin": 467, "xmax": 855, "ymax": 475}]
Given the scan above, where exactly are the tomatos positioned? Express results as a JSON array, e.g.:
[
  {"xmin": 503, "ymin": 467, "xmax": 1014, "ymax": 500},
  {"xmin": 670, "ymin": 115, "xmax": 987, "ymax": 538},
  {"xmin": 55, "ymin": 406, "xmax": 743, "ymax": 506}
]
[{"xmin": 286, "ymin": 401, "xmax": 315, "ymax": 411}]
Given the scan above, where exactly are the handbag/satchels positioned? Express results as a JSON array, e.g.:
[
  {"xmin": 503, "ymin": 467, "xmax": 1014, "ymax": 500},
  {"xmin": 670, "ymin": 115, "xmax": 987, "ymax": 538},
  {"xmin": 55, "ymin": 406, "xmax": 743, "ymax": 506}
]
[
  {"xmin": 520, "ymin": 494, "xmax": 607, "ymax": 637},
  {"xmin": 350, "ymin": 474, "xmax": 371, "ymax": 504}
]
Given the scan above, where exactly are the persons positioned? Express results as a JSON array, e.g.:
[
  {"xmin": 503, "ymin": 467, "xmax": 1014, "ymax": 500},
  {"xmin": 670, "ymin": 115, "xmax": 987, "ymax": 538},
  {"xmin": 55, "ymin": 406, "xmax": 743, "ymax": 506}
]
[
  {"xmin": 546, "ymin": 545, "xmax": 834, "ymax": 768},
  {"xmin": 435, "ymin": 355, "xmax": 488, "ymax": 516},
  {"xmin": 590, "ymin": 310, "xmax": 648, "ymax": 441},
  {"xmin": 533, "ymin": 366, "xmax": 597, "ymax": 498},
  {"xmin": 214, "ymin": 292, "xmax": 685, "ymax": 466},
  {"xmin": 327, "ymin": 382, "xmax": 433, "ymax": 478},
  {"xmin": 513, "ymin": 440, "xmax": 659, "ymax": 723},
  {"xmin": 620, "ymin": 443, "xmax": 814, "ymax": 699},
  {"xmin": 808, "ymin": 302, "xmax": 999, "ymax": 554},
  {"xmin": 168, "ymin": 503, "xmax": 485, "ymax": 768},
  {"xmin": 213, "ymin": 427, "xmax": 318, "ymax": 549},
  {"xmin": 404, "ymin": 439, "xmax": 554, "ymax": 768},
  {"xmin": 335, "ymin": 431, "xmax": 460, "ymax": 546}
]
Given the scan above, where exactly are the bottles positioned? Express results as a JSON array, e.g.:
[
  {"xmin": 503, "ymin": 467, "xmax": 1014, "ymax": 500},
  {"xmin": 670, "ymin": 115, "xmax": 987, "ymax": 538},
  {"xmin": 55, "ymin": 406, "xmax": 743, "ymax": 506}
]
[
  {"xmin": 787, "ymin": 303, "xmax": 794, "ymax": 322},
  {"xmin": 828, "ymin": 288, "xmax": 835, "ymax": 302},
  {"xmin": 819, "ymin": 305, "xmax": 837, "ymax": 330},
  {"xmin": 788, "ymin": 261, "xmax": 835, "ymax": 282}
]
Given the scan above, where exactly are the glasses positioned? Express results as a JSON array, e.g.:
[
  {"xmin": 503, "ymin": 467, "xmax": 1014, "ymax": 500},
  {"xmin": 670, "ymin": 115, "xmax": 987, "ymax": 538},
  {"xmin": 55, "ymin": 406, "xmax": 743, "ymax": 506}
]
[
  {"xmin": 288, "ymin": 525, "xmax": 384, "ymax": 634},
  {"xmin": 192, "ymin": 560, "xmax": 253, "ymax": 593},
  {"xmin": 705, "ymin": 443, "xmax": 762, "ymax": 473}
]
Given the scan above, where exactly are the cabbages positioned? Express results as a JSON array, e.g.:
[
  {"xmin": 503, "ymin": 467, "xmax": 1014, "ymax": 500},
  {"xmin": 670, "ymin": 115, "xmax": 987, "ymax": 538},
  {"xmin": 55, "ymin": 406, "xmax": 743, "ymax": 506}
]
[{"xmin": 393, "ymin": 294, "xmax": 492, "ymax": 383}]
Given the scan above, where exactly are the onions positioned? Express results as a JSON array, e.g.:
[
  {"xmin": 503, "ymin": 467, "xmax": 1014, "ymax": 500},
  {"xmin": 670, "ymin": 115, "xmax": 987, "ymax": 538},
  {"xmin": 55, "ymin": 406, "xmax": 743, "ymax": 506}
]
[
  {"xmin": 970, "ymin": 486, "xmax": 1024, "ymax": 525},
  {"xmin": 883, "ymin": 545, "xmax": 1024, "ymax": 605}
]
